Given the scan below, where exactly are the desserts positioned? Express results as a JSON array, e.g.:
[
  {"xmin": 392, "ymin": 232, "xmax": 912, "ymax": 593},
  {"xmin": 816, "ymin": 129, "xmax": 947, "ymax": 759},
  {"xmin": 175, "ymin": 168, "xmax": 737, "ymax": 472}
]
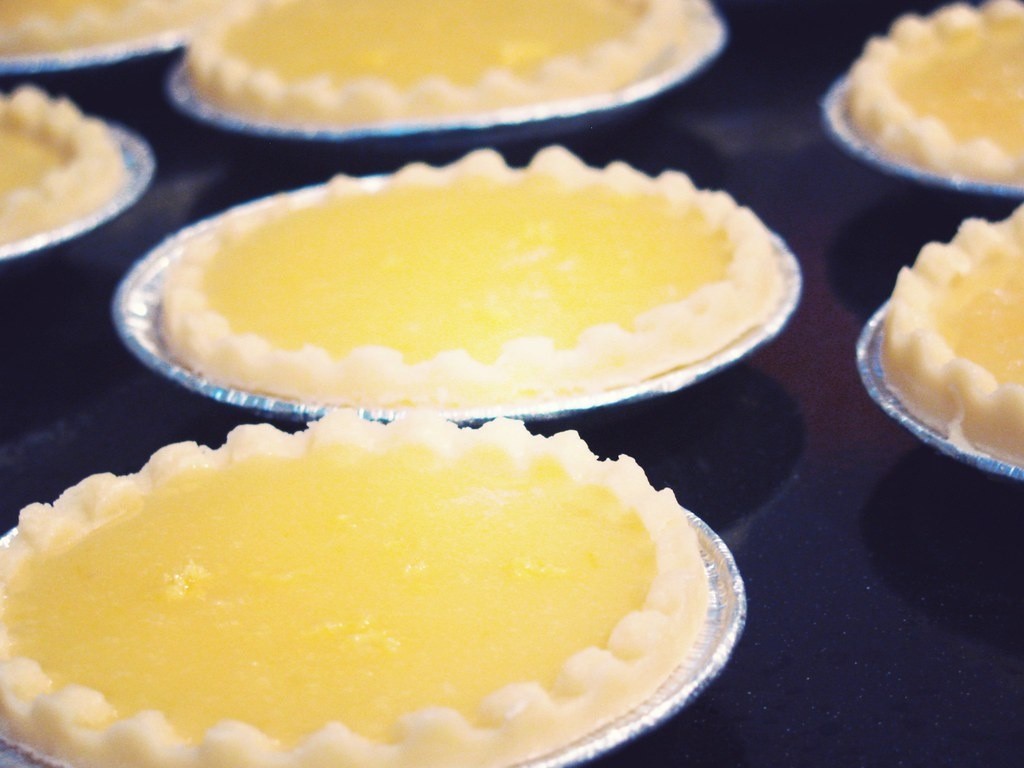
[{"xmin": 0, "ymin": 0, "xmax": 1024, "ymax": 768}]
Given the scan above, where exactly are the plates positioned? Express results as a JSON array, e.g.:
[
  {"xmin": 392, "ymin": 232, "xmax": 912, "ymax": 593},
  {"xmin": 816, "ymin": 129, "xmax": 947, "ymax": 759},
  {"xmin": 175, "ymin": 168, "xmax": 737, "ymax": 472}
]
[
  {"xmin": 0, "ymin": 22, "xmax": 191, "ymax": 79},
  {"xmin": 855, "ymin": 297, "xmax": 1024, "ymax": 483},
  {"xmin": 0, "ymin": 116, "xmax": 156, "ymax": 264},
  {"xmin": 110, "ymin": 174, "xmax": 802, "ymax": 429},
  {"xmin": 164, "ymin": 14, "xmax": 728, "ymax": 143},
  {"xmin": 0, "ymin": 502, "xmax": 745, "ymax": 768},
  {"xmin": 818, "ymin": 75, "xmax": 1024, "ymax": 199}
]
